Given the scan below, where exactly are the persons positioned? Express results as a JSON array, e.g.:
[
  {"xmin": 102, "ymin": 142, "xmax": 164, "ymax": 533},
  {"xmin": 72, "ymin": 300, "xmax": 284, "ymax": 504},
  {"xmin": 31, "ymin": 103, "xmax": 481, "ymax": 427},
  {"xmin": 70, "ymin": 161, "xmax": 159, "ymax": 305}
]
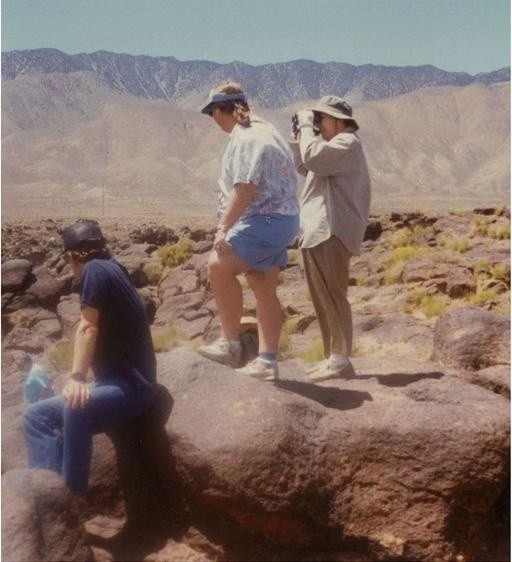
[
  {"xmin": 21, "ymin": 217, "xmax": 159, "ymax": 497},
  {"xmin": 289, "ymin": 94, "xmax": 372, "ymax": 382},
  {"xmin": 199, "ymin": 80, "xmax": 301, "ymax": 382},
  {"xmin": 15, "ymin": 354, "xmax": 55, "ymax": 404}
]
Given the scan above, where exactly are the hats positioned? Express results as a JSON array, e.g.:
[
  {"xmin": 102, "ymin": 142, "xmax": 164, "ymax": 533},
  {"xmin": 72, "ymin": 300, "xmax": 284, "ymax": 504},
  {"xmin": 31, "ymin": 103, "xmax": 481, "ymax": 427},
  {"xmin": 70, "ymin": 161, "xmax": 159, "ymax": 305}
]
[
  {"xmin": 58, "ymin": 220, "xmax": 103, "ymax": 259},
  {"xmin": 201, "ymin": 90, "xmax": 246, "ymax": 115},
  {"xmin": 308, "ymin": 95, "xmax": 359, "ymax": 130}
]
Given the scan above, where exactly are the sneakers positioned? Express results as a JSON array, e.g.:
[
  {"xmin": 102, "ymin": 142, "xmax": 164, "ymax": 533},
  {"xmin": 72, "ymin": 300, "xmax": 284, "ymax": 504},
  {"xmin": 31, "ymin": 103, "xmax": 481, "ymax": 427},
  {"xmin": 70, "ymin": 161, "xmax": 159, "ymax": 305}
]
[
  {"xmin": 307, "ymin": 357, "xmax": 328, "ymax": 374},
  {"xmin": 307, "ymin": 360, "xmax": 354, "ymax": 383},
  {"xmin": 197, "ymin": 337, "xmax": 244, "ymax": 367},
  {"xmin": 235, "ymin": 357, "xmax": 279, "ymax": 385}
]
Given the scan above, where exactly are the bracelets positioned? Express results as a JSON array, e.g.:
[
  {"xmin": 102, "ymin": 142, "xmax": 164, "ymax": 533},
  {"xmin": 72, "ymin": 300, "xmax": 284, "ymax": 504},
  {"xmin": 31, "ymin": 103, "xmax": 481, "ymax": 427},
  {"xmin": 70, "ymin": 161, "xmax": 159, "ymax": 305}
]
[{"xmin": 215, "ymin": 225, "xmax": 226, "ymax": 233}]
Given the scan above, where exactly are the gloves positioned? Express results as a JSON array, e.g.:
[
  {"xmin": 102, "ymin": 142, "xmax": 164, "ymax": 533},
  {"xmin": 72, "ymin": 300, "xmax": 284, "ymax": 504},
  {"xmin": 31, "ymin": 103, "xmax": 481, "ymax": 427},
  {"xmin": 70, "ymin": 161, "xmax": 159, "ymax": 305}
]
[{"xmin": 298, "ymin": 111, "xmax": 314, "ymax": 130}]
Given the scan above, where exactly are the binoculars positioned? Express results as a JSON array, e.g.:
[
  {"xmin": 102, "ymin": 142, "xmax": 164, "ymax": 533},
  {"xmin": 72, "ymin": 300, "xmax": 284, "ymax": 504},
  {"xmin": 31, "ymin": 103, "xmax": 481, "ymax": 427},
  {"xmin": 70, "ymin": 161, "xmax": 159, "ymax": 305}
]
[{"xmin": 292, "ymin": 114, "xmax": 321, "ymax": 124}]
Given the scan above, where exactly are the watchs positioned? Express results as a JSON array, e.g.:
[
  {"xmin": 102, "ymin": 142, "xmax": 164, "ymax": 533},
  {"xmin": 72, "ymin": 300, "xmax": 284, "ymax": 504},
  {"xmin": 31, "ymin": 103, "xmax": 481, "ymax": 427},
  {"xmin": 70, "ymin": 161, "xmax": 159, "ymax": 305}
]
[{"xmin": 70, "ymin": 372, "xmax": 88, "ymax": 383}]
